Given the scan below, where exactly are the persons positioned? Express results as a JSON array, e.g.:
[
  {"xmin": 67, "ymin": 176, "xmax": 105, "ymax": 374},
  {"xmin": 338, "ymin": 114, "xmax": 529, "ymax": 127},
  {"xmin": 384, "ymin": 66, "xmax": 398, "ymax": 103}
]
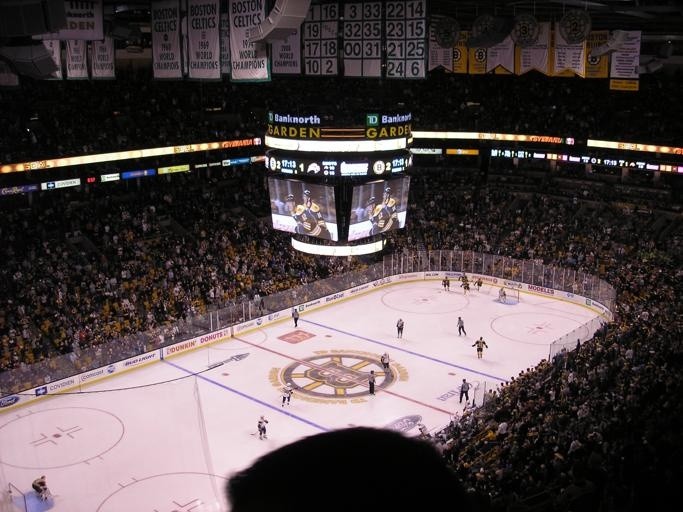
[{"xmin": 0, "ymin": 78, "xmax": 682, "ymax": 512}]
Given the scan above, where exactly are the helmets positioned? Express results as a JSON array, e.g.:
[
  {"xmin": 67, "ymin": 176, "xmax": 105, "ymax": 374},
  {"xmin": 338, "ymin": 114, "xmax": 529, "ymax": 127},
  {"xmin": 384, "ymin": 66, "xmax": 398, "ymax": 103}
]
[
  {"xmin": 303, "ymin": 189, "xmax": 312, "ymax": 196},
  {"xmin": 385, "ymin": 187, "xmax": 392, "ymax": 194},
  {"xmin": 365, "ymin": 197, "xmax": 378, "ymax": 206},
  {"xmin": 284, "ymin": 194, "xmax": 296, "ymax": 201}
]
